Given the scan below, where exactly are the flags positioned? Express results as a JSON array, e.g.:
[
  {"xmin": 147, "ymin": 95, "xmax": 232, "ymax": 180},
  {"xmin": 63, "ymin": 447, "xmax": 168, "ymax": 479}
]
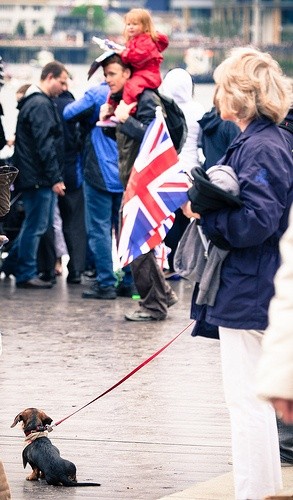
[{"xmin": 119, "ymin": 115, "xmax": 190, "ymax": 271}]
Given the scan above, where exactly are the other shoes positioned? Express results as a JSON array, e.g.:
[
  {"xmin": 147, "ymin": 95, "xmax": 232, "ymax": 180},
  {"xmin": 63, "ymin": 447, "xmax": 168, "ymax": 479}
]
[
  {"xmin": 110, "ymin": 116, "xmax": 121, "ymax": 123},
  {"xmin": 125, "ymin": 311, "xmax": 154, "ymax": 321},
  {"xmin": 43, "ymin": 275, "xmax": 56, "ymax": 284},
  {"xmin": 116, "ymin": 287, "xmax": 138, "ymax": 297},
  {"xmin": 82, "ymin": 290, "xmax": 116, "ymax": 299},
  {"xmin": 1, "ymin": 261, "xmax": 17, "ymax": 278},
  {"xmin": 162, "ymin": 269, "xmax": 181, "ymax": 282},
  {"xmin": 66, "ymin": 274, "xmax": 81, "ymax": 284},
  {"xmin": 16, "ymin": 277, "xmax": 51, "ymax": 289},
  {"xmin": 277, "ymin": 417, "xmax": 293, "ymax": 463},
  {"xmin": 96, "ymin": 119, "xmax": 117, "ymax": 128},
  {"xmin": 167, "ymin": 294, "xmax": 177, "ymax": 307},
  {"xmin": 86, "ymin": 271, "xmax": 99, "ymax": 281}
]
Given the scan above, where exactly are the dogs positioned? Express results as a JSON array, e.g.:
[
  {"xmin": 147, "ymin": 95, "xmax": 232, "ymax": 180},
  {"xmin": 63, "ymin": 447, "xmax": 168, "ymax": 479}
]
[{"xmin": 10, "ymin": 408, "xmax": 101, "ymax": 486}]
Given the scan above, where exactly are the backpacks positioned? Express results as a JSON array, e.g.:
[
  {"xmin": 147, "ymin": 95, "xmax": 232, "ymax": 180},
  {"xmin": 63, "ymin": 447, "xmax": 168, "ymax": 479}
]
[{"xmin": 137, "ymin": 88, "xmax": 187, "ymax": 155}]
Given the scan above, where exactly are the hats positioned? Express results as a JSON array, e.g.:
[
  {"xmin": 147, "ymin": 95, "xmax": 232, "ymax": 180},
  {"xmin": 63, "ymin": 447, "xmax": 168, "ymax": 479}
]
[{"xmin": 186, "ymin": 167, "xmax": 245, "ymax": 218}]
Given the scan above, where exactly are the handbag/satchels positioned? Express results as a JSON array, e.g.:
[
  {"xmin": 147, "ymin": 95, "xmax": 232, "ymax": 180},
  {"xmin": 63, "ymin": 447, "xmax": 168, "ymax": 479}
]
[{"xmin": 0, "ymin": 166, "xmax": 19, "ymax": 216}]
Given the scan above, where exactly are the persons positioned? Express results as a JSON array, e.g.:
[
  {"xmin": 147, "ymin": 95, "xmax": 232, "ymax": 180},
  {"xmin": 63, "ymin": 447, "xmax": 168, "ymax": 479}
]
[
  {"xmin": 13, "ymin": 62, "xmax": 98, "ymax": 290},
  {"xmin": 158, "ymin": 69, "xmax": 203, "ymax": 280},
  {"xmin": 180, "ymin": 47, "xmax": 293, "ymax": 500},
  {"xmin": 63, "ymin": 53, "xmax": 139, "ymax": 300},
  {"xmin": 257, "ymin": 194, "xmax": 293, "ymax": 429},
  {"xmin": 100, "ymin": 54, "xmax": 178, "ymax": 325},
  {"xmin": 98, "ymin": 7, "xmax": 168, "ymax": 126}
]
